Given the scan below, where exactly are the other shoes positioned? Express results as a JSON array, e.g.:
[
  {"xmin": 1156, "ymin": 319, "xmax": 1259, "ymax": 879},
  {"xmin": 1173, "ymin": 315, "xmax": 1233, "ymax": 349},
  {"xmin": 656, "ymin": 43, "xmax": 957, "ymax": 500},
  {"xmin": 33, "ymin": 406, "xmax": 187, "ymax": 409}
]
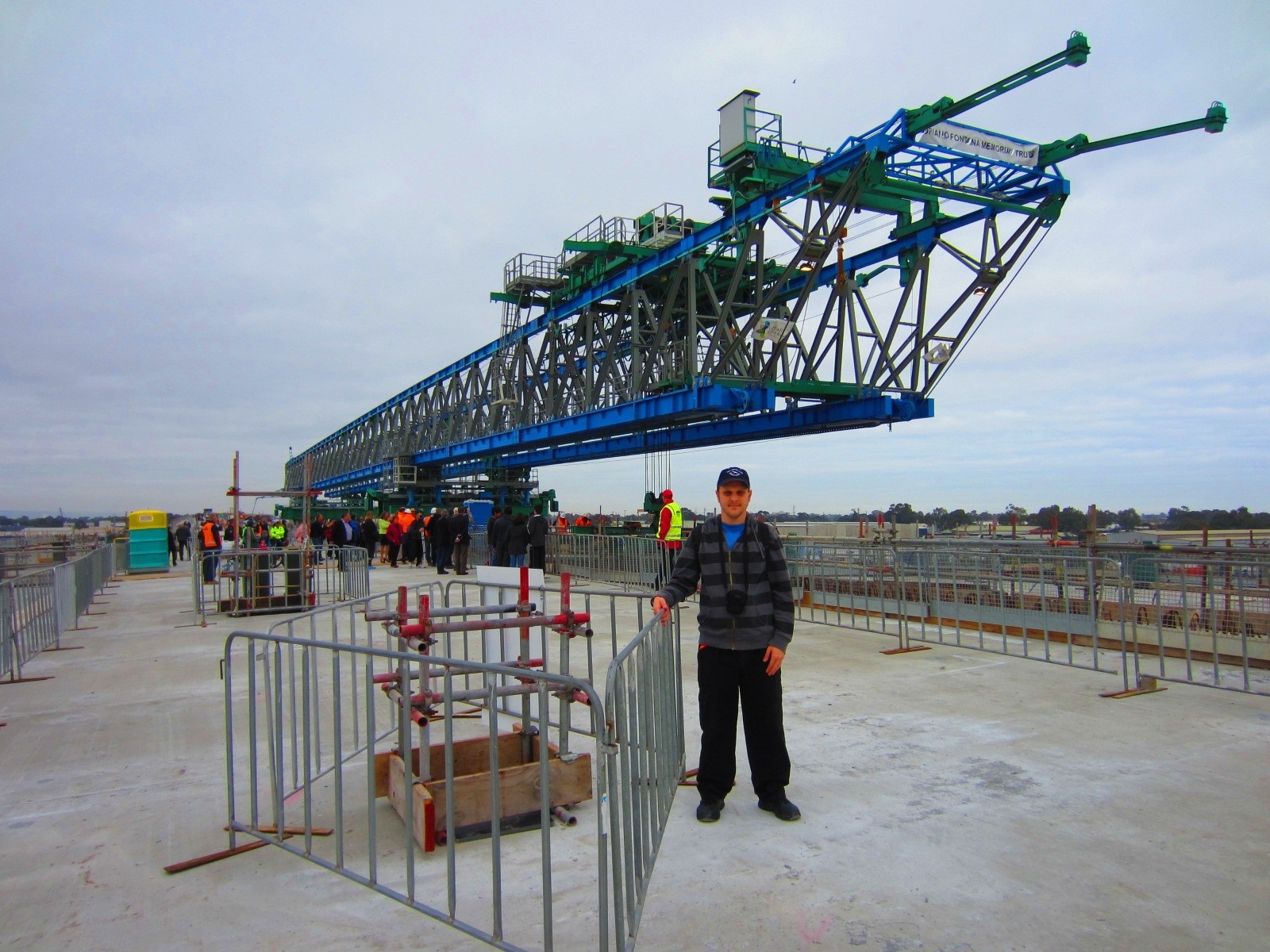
[
  {"xmin": 204, "ymin": 579, "xmax": 221, "ymax": 585},
  {"xmin": 369, "ymin": 558, "xmax": 469, "ymax": 575},
  {"xmin": 312, "ymin": 556, "xmax": 333, "ymax": 565},
  {"xmin": 338, "ymin": 568, "xmax": 349, "ymax": 572},
  {"xmin": 648, "ymin": 583, "xmax": 659, "ymax": 591}
]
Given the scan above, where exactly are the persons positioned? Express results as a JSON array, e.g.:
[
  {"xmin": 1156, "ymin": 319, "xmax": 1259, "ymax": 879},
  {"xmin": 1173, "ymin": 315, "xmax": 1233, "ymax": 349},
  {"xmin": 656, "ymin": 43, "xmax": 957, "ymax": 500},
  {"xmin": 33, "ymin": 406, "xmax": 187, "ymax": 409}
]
[
  {"xmin": 174, "ymin": 520, "xmax": 194, "ymax": 561},
  {"xmin": 486, "ymin": 504, "xmax": 548, "ymax": 579},
  {"xmin": 378, "ymin": 506, "xmax": 472, "ymax": 576},
  {"xmin": 168, "ymin": 526, "xmax": 178, "ymax": 566},
  {"xmin": 574, "ymin": 512, "xmax": 593, "ymax": 526},
  {"xmin": 199, "ymin": 513, "xmax": 221, "ymax": 585},
  {"xmin": 223, "ymin": 515, "xmax": 310, "ymax": 565},
  {"xmin": 311, "ymin": 512, "xmax": 378, "ymax": 570},
  {"xmin": 552, "ymin": 513, "xmax": 570, "ymax": 533},
  {"xmin": 651, "ymin": 468, "xmax": 804, "ymax": 821},
  {"xmin": 650, "ymin": 489, "xmax": 681, "ymax": 592}
]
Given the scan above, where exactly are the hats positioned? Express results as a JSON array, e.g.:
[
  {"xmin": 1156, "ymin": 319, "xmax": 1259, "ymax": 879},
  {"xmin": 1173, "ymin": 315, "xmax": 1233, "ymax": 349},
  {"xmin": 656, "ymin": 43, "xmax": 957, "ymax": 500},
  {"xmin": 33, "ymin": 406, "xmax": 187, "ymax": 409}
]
[
  {"xmin": 717, "ymin": 467, "xmax": 751, "ymax": 487},
  {"xmin": 246, "ymin": 520, "xmax": 255, "ymax": 527},
  {"xmin": 661, "ymin": 490, "xmax": 672, "ymax": 498},
  {"xmin": 272, "ymin": 519, "xmax": 281, "ymax": 525}
]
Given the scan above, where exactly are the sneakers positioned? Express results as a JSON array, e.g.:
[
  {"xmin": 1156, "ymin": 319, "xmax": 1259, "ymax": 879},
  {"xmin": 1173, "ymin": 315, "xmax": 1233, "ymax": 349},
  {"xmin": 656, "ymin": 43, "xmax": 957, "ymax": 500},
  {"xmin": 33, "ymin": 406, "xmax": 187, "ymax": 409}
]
[
  {"xmin": 697, "ymin": 799, "xmax": 725, "ymax": 823},
  {"xmin": 758, "ymin": 800, "xmax": 801, "ymax": 821}
]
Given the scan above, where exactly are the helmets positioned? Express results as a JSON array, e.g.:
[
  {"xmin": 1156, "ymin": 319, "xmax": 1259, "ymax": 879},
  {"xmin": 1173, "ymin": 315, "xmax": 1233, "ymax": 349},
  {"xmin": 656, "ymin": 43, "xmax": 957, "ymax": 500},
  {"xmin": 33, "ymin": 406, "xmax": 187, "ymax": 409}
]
[
  {"xmin": 404, "ymin": 508, "xmax": 412, "ymax": 514},
  {"xmin": 184, "ymin": 520, "xmax": 191, "ymax": 526},
  {"xmin": 430, "ymin": 508, "xmax": 438, "ymax": 514},
  {"xmin": 206, "ymin": 512, "xmax": 218, "ymax": 522}
]
